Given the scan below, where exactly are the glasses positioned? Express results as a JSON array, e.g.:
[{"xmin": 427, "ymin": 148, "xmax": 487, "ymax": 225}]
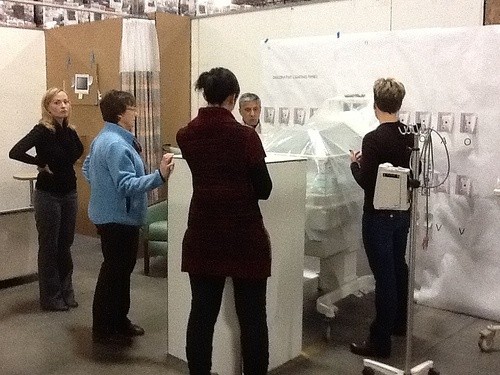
[{"xmin": 126, "ymin": 107, "xmax": 138, "ymax": 113}]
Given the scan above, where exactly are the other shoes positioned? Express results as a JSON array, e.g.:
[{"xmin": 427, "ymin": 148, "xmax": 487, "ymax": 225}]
[
  {"xmin": 393, "ymin": 327, "xmax": 407, "ymax": 336},
  {"xmin": 55, "ymin": 305, "xmax": 68, "ymax": 312},
  {"xmin": 350, "ymin": 341, "xmax": 383, "ymax": 358},
  {"xmin": 131, "ymin": 323, "xmax": 145, "ymax": 337},
  {"xmin": 67, "ymin": 301, "xmax": 78, "ymax": 308}
]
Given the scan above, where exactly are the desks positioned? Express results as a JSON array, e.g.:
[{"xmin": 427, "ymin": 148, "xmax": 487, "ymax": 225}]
[{"xmin": 13, "ymin": 169, "xmax": 39, "ymax": 208}]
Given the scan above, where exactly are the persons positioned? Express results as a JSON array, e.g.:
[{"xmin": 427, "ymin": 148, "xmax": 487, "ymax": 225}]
[
  {"xmin": 239, "ymin": 92, "xmax": 275, "ymax": 135},
  {"xmin": 350, "ymin": 77, "xmax": 421, "ymax": 365},
  {"xmin": 9, "ymin": 86, "xmax": 85, "ymax": 311},
  {"xmin": 175, "ymin": 68, "xmax": 271, "ymax": 375},
  {"xmin": 81, "ymin": 90, "xmax": 175, "ymax": 337}
]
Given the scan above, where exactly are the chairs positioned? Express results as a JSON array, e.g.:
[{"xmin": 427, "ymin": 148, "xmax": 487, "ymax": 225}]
[{"xmin": 141, "ymin": 199, "xmax": 167, "ymax": 273}]
